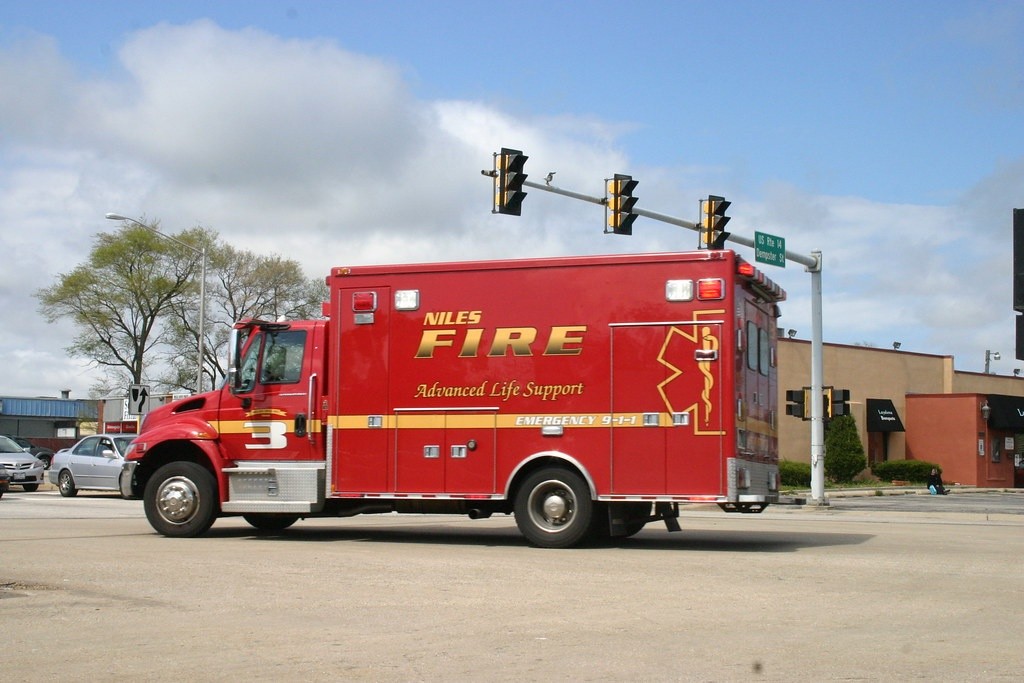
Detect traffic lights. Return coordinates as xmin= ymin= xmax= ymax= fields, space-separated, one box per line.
xmin=784 ymin=389 xmax=804 ymax=419
xmin=610 ymin=173 xmax=640 ymax=235
xmin=703 ymin=194 xmax=731 ymax=248
xmin=832 ymin=389 xmax=850 ymax=418
xmin=500 ymin=148 xmax=529 ymax=215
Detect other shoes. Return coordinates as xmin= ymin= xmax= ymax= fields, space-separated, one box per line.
xmin=943 ymin=490 xmax=950 ymax=494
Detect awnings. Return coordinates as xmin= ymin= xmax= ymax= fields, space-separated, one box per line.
xmin=866 ymin=398 xmax=906 ymax=432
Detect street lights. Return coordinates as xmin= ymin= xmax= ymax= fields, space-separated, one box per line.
xmin=105 ymin=213 xmax=205 ymax=395
xmin=985 ymin=352 xmax=1000 ymax=373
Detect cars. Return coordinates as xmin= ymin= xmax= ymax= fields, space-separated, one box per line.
xmin=5 ymin=435 xmax=56 ymax=469
xmin=48 ymin=434 xmax=139 ymax=499
xmin=0 ymin=435 xmax=46 ymax=493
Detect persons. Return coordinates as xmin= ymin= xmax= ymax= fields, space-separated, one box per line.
xmin=928 ymin=468 xmax=950 ymax=495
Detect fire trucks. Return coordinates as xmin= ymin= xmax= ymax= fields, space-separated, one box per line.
xmin=118 ymin=249 xmax=787 ymax=546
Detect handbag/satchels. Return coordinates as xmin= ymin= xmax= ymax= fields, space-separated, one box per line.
xmin=929 ymin=485 xmax=937 ymax=494
